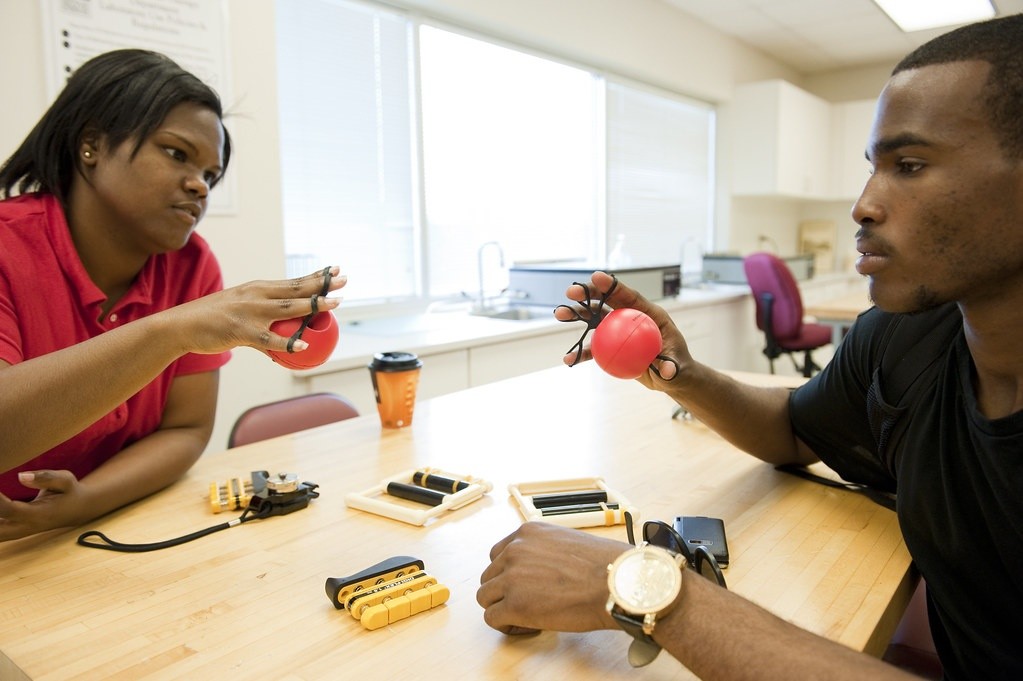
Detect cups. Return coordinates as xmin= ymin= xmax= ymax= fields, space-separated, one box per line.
xmin=368 ymin=351 xmax=424 ymax=430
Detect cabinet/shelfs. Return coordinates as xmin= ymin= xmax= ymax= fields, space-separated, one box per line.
xmin=732 ymin=80 xmax=883 ymax=198
xmin=309 ymin=302 xmax=740 ymax=416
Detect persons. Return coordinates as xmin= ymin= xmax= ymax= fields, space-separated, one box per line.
xmin=477 ymin=9 xmax=1023 ymax=679
xmin=0 ymin=48 xmax=347 ymax=542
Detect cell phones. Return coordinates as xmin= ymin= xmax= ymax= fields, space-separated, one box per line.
xmin=672 ymin=516 xmax=729 ymax=569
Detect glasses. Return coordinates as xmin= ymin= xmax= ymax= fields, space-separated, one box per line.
xmin=625 ymin=511 xmax=728 ymax=590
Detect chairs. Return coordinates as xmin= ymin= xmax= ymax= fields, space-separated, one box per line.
xmin=744 ymin=253 xmax=833 ymax=378
xmin=229 ymin=391 xmax=360 ymax=446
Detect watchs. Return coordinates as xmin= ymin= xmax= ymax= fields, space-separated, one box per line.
xmin=605 ymin=541 xmax=689 ymax=668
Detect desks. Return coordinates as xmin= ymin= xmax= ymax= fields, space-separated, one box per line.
xmin=0 ymin=359 xmax=921 ymax=681
xmin=792 ymin=279 xmax=874 ymax=318
